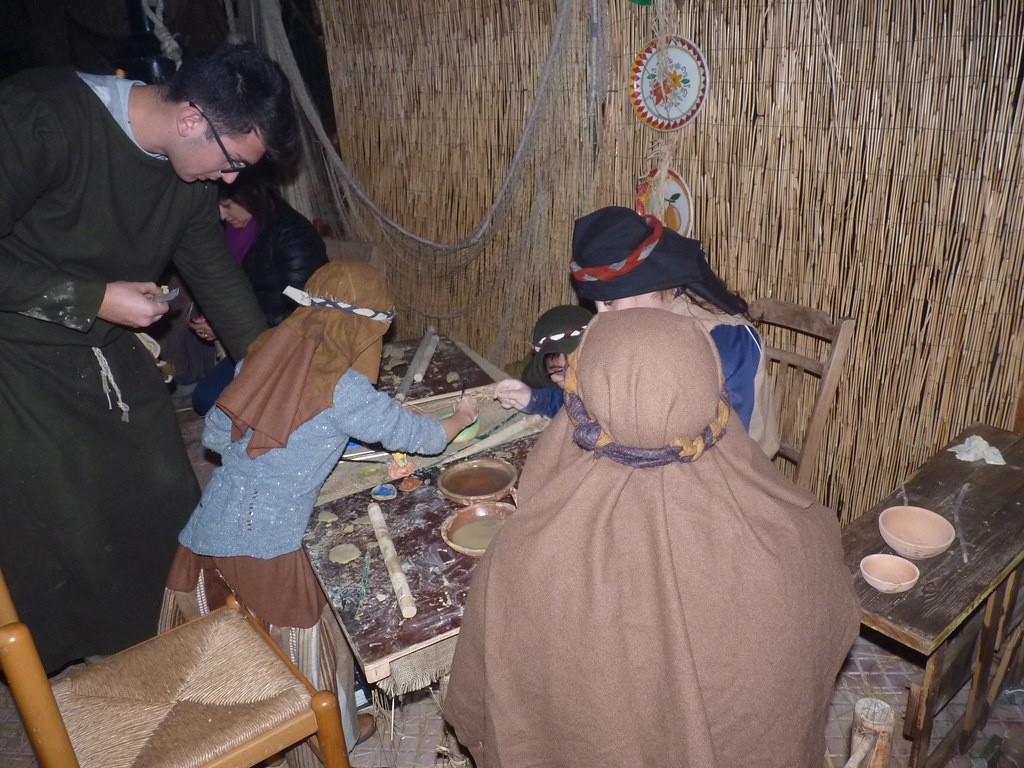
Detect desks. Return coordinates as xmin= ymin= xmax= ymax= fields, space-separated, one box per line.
xmin=839 ymin=421 xmax=1024 ymax=768
xmin=299 ymin=337 xmax=552 ymax=709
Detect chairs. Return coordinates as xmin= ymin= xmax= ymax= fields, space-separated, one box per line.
xmin=0 ymin=576 xmax=352 ymax=768
xmin=748 ymin=299 xmax=857 ymax=488
xmin=215 ymin=238 xmax=384 ymax=360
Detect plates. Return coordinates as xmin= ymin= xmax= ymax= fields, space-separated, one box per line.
xmin=637 ymin=168 xmax=694 ymax=238
xmin=629 ymin=35 xmax=709 ymax=131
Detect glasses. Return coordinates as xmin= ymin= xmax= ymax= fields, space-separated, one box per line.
xmin=544 ymin=369 xmax=566 ymax=383
xmin=189 ymin=102 xmax=249 ymax=173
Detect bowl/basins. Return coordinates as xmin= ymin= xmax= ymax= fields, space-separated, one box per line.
xmin=439 ymin=413 xmax=480 ymax=442
xmin=860 ymin=554 xmax=920 ymax=594
xmin=878 ymin=506 xmax=956 ymax=561
xmin=441 ymin=501 xmax=517 ymax=557
xmin=438 ymin=457 xmax=518 ymax=506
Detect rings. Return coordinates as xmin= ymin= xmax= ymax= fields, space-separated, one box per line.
xmin=203 ymin=333 xmax=208 ymax=337
xmin=203 ymin=317 xmax=206 ymax=321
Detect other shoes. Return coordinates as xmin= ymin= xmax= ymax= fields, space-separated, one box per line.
xmin=354 ymin=712 xmax=376 ymax=746
xmin=266 ymin=752 xmax=284 ymax=766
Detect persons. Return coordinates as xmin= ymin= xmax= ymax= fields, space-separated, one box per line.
xmin=158 ymin=259 xmax=479 ymax=768
xmin=569 ymin=206 xmax=780 ymax=461
xmin=443 ymin=307 xmax=862 ymax=768
xmin=188 ymin=170 xmax=330 ymax=416
xmin=1 ymin=47 xmax=303 ymax=683
xmin=493 ymin=305 xmax=593 ymax=419
